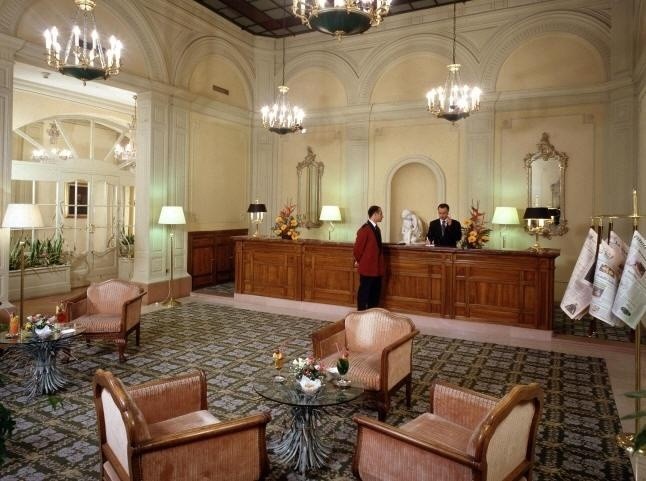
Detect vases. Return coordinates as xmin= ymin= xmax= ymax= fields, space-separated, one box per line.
xmin=33 ymin=325 xmax=51 ymax=338
xmin=298 ymin=374 xmax=321 ymax=391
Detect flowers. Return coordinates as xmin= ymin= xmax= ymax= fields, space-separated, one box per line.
xmin=289 ymin=356 xmax=328 ymax=378
xmin=458 ymin=199 xmax=492 ymax=250
xmin=24 ymin=310 xmax=57 ymax=337
xmin=270 ymin=199 xmax=303 ymax=240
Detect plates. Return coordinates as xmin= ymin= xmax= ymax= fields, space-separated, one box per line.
xmin=412 ymin=243 xmax=426 ymax=247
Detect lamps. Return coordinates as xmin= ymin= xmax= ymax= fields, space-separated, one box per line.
xmin=490 ymin=204 xmax=520 ymax=250
xmin=39 ymin=0 xmax=126 ymax=88
xmin=155 ymin=205 xmax=191 ymax=309
xmin=256 ymin=25 xmax=308 ymax=136
xmin=289 ymin=0 xmax=399 ymax=39
xmin=246 ymin=202 xmax=268 ymax=238
xmin=524 ymin=205 xmax=553 ymax=252
xmin=417 ymin=0 xmax=485 ymax=127
xmin=30 ymin=117 xmax=74 ymax=165
xmin=319 ymin=205 xmax=343 ymax=240
xmin=111 ymin=94 xmax=141 ymax=169
xmin=1 ymin=201 xmax=49 ymax=328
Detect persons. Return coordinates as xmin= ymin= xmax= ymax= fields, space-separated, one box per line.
xmin=428 ymin=203 xmax=461 ymax=248
xmin=352 ymin=203 xmax=386 ymax=312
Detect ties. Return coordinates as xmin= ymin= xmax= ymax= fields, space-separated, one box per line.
xmin=441 ymin=221 xmax=446 ymax=237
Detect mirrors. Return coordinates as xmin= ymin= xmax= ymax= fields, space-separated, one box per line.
xmin=295 ymin=145 xmax=325 ymax=231
xmin=525 ymin=131 xmax=569 ymax=243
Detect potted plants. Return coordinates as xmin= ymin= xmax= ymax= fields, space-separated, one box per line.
xmin=117 ymin=231 xmax=134 ymax=281
xmin=6 ymin=233 xmax=70 ymax=304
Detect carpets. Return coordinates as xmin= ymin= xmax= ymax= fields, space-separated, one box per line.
xmin=553 ymin=301 xmax=644 ymax=350
xmin=193 ymin=277 xmax=234 ymax=297
xmin=1 ymin=297 xmax=638 ymax=480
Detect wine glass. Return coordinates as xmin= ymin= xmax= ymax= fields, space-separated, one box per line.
xmin=335 ymin=356 xmax=352 ymax=389
xmin=270 ymin=353 xmax=285 ymax=383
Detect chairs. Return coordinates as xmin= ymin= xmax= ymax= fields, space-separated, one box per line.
xmin=305 ymin=305 xmax=425 ymax=423
xmin=348 ymin=365 xmax=545 ymax=481
xmin=52 ymin=278 xmax=147 ymax=368
xmin=84 ymin=362 xmax=272 ymax=480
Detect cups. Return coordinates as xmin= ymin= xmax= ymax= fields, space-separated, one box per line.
xmin=9 ymin=316 xmax=20 ymax=337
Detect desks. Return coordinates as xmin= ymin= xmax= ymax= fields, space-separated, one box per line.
xmin=2 ymin=322 xmax=88 ymax=402
xmin=244 ymin=361 xmax=370 ymax=477
